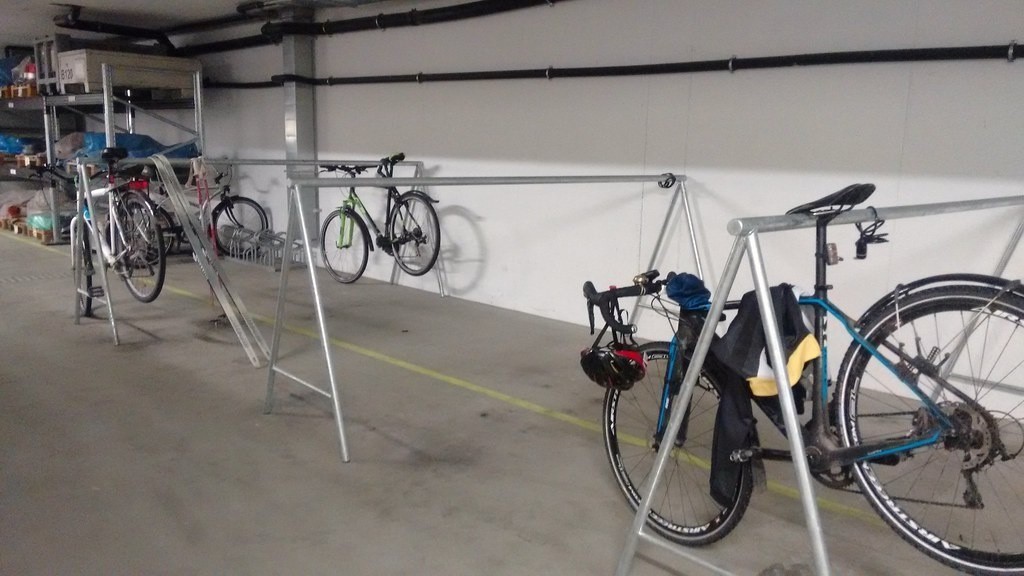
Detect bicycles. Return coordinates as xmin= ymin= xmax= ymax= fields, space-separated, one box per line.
xmin=107 ymin=155 xmax=270 ymax=269
xmin=27 ymin=146 xmax=166 ymax=315
xmin=582 ymin=184 xmax=1024 ymax=576
xmin=318 ymin=153 xmax=442 ymax=283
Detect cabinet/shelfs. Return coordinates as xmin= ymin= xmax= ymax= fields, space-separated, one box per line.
xmin=0 ymin=65 xmax=211 ymax=244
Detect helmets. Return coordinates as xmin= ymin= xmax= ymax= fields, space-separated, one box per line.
xmin=580 ymin=343 xmax=648 ymax=391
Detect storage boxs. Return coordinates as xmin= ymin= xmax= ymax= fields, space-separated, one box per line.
xmin=17 ymin=153 xmax=37 ymax=168
xmin=2 ymin=218 xmax=53 ymax=241
xmin=66 ymin=162 xmax=97 ymax=177
xmin=57 ymin=49 xmax=202 ymax=98
xmin=0 ymin=85 xmax=36 ymax=99
